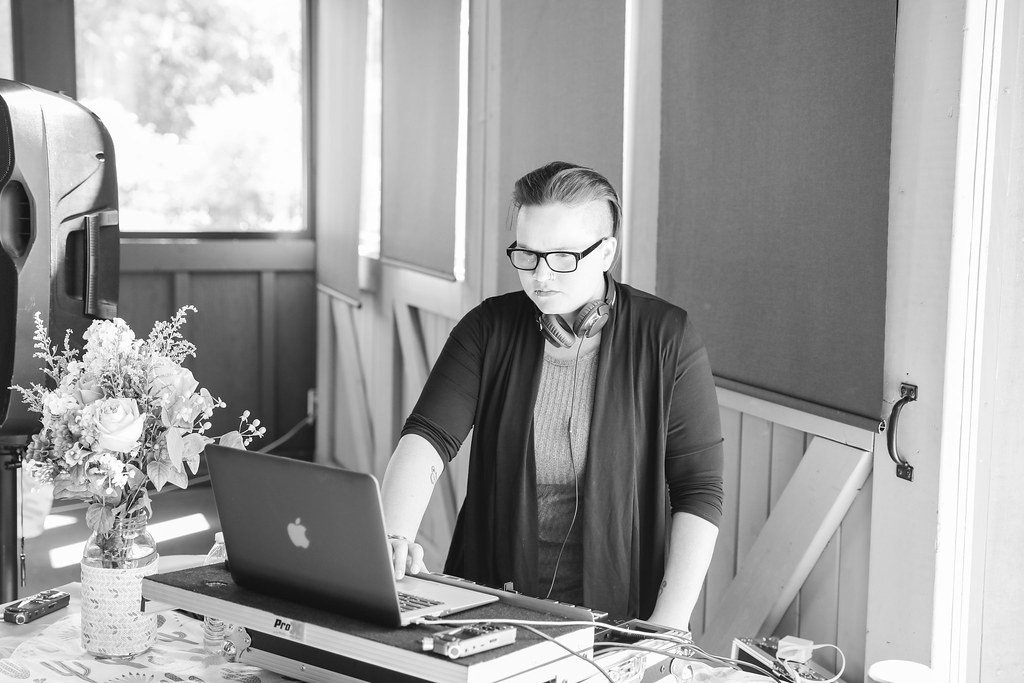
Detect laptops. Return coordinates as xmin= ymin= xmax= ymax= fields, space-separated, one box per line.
xmin=205 ymin=443 xmax=499 ymax=627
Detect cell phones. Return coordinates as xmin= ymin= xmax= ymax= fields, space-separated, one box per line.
xmin=426 ymin=623 xmax=516 ymax=660
xmin=4 ymin=591 xmax=70 ymax=624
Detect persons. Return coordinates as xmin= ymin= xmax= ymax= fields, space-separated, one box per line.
xmin=381 ymin=161 xmax=723 ymax=633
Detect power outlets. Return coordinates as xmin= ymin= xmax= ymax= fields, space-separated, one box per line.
xmin=307 ymin=389 xmax=318 ymax=422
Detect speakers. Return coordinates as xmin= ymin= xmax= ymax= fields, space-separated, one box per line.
xmin=0 ymin=77 xmax=120 ymax=441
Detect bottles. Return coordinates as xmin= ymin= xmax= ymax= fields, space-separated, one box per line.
xmin=201 ymin=532 xmax=230 ymax=654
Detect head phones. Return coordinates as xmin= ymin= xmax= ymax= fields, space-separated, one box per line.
xmin=534 ymin=270 xmax=616 ymax=348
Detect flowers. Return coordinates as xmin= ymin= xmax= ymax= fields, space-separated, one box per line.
xmin=8 ymin=302 xmax=264 ymax=533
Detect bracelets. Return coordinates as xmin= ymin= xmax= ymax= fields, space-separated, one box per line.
xmin=387 ymin=533 xmax=406 ymax=539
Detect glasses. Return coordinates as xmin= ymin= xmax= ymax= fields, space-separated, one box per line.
xmin=506 ymin=237 xmax=607 ymax=273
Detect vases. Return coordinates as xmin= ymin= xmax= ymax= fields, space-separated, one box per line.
xmin=81 ymin=510 xmax=160 ymax=659
